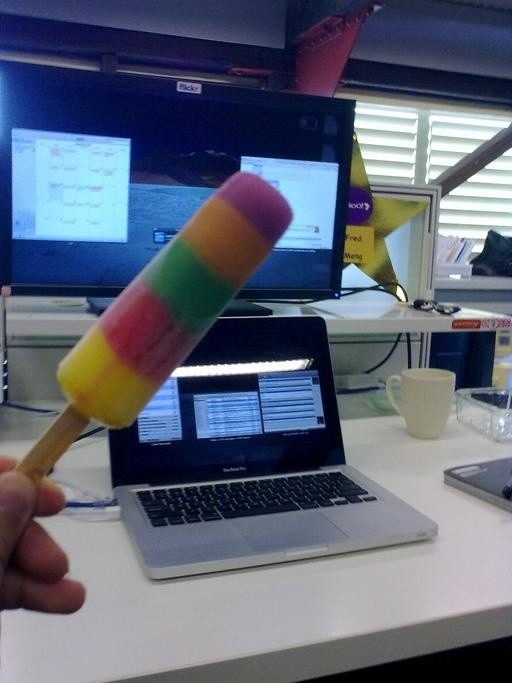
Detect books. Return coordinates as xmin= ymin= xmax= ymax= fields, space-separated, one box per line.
xmin=443 ymin=457 xmax=512 ymax=514
xmin=435 ymin=234 xmax=477 ymax=264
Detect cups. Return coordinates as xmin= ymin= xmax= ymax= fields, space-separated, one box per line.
xmin=387 ymin=367 xmax=459 ymax=438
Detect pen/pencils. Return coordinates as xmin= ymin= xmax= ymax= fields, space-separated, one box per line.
xmin=502 ymin=471 xmax=512 ymax=496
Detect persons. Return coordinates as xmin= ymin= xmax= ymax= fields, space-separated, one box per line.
xmin=1 ymin=458 xmax=86 ymax=615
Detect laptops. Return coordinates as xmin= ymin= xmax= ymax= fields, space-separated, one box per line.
xmin=108 ymin=314 xmax=441 ymax=581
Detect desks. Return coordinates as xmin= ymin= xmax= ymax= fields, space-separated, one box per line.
xmin=1 ymin=289 xmax=512 ymax=683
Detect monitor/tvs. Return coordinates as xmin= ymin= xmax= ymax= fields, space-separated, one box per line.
xmin=1 ymin=57 xmax=357 ymax=315
